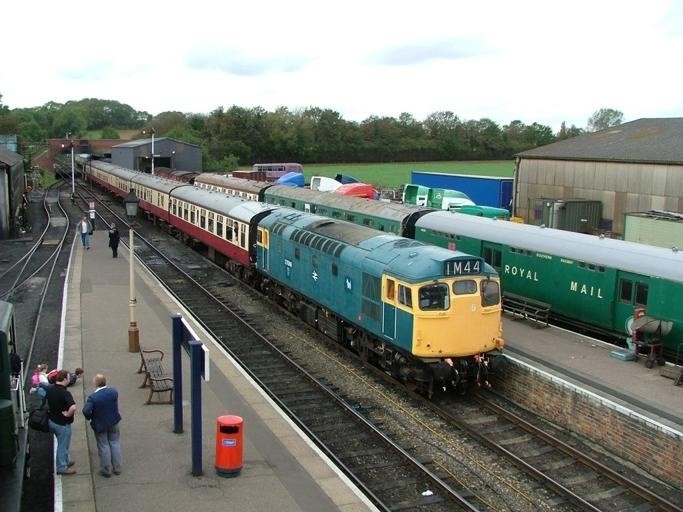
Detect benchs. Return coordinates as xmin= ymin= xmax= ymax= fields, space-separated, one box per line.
xmin=137 ymin=344 xmax=174 ymax=405
xmin=501 ymin=291 xmax=552 ymax=329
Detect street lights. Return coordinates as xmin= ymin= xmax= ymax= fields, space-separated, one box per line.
xmin=124 ymin=188 xmax=144 ymax=353
xmin=70 ymin=141 xmax=78 ymax=205
xmin=149 ymin=128 xmax=160 ymax=177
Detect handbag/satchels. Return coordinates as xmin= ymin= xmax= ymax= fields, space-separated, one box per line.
xmin=28 ymin=408 xmax=50 ymax=433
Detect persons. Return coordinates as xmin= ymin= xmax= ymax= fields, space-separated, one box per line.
xmin=106 ymin=222 xmax=119 ymax=259
xmin=75 ymin=215 xmax=93 ymax=249
xmin=32 ymin=363 xmax=49 ymax=412
xmin=42 ymin=369 xmax=77 ymax=478
xmin=25 ymin=367 xmax=83 ymax=396
xmin=80 ymin=373 xmax=122 ymax=479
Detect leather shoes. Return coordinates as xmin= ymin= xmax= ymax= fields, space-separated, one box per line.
xmin=57 ymin=461 xmax=77 ymax=474
xmin=99 ymin=470 xmax=121 ymax=477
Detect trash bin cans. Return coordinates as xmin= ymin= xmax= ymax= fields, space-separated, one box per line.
xmin=214 ymin=414 xmax=243 ymax=478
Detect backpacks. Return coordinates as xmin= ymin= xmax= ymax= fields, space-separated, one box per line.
xmin=47 ymin=369 xmax=59 ymax=384
xmin=32 ymin=372 xmax=39 ymax=385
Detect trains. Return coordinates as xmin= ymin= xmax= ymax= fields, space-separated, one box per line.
xmin=81 ymin=160 xmax=509 ymax=401
xmin=0 ymin=299 xmax=29 ymax=468
xmin=146 ymin=164 xmax=683 ymax=369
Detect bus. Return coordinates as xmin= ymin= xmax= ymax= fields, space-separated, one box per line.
xmin=251 ymin=162 xmax=304 ymax=183
